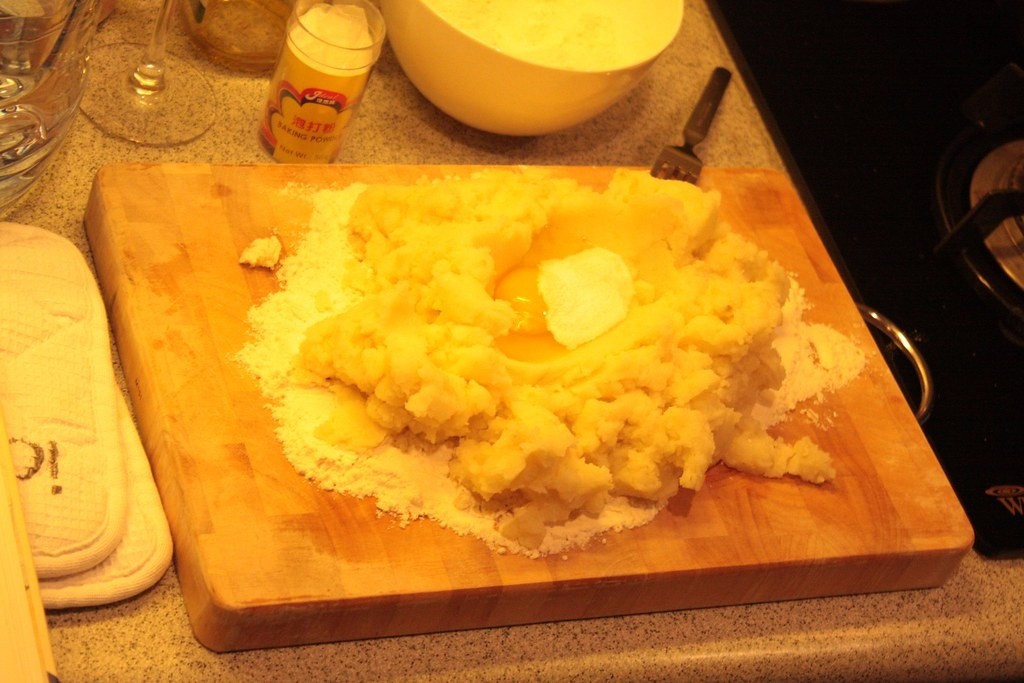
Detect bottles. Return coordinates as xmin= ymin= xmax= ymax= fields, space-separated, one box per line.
xmin=257 ymin=1 xmax=387 ymax=168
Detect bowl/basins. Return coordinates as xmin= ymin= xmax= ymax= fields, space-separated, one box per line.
xmin=382 ymin=0 xmax=683 ymax=138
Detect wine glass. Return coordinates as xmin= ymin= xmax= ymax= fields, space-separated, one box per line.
xmin=78 ymin=1 xmax=221 ymax=147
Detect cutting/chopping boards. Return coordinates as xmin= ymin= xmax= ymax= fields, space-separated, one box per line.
xmin=83 ymin=160 xmax=975 ymax=654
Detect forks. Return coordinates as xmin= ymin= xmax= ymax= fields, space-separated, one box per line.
xmin=649 ymin=67 xmax=731 ymax=182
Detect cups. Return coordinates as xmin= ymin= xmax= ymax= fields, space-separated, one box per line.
xmin=0 ymin=1 xmax=99 ymax=220
xmin=175 ymin=1 xmax=293 ymax=74
